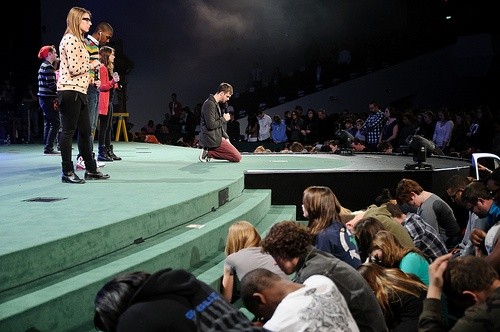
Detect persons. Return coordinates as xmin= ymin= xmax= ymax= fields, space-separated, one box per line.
xmin=199 ymin=83 xmax=242 ymax=163
xmin=0 ymin=7 xmax=500 ymax=332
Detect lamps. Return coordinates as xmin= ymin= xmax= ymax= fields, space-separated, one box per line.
xmin=404 ymin=134 xmax=436 ymax=171
xmin=335 ymin=130 xmax=355 ymax=155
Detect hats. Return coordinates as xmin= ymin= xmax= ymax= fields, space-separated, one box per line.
xmin=227 ymin=106 xmax=234 ymax=113
xmin=38 ymin=46 xmax=51 ymax=59
xmin=485 ymin=224 xmax=500 ymax=256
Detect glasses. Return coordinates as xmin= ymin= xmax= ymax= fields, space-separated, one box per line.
xmin=450 ymin=189 xmax=460 ymax=203
xmin=82 ymin=18 xmax=92 ymax=21
xmin=466 ymin=204 xmax=475 ymax=215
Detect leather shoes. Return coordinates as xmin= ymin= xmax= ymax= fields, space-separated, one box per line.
xmin=62 ymin=170 xmax=86 ymax=184
xmin=85 ymin=170 xmax=110 ymax=180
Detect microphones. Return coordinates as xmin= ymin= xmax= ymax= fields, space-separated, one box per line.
xmin=94 ymin=66 xmax=99 ymax=88
xmin=225 ymin=102 xmax=228 ymax=113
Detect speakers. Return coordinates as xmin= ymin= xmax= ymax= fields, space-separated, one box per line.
xmin=413 ymin=137 xmax=428 ymax=162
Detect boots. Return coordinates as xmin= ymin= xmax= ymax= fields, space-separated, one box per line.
xmin=97 ymin=144 xmax=113 ymax=161
xmin=105 ymin=145 xmax=121 ymax=160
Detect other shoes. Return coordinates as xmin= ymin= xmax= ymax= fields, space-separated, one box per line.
xmin=199 ymin=148 xmax=210 ymax=163
xmin=57 ymin=145 xmax=74 ymax=151
xmin=96 ymin=162 xmax=105 ymax=168
xmin=76 ymin=156 xmax=86 ymax=171
xmin=43 ymin=150 xmax=61 ymax=155
